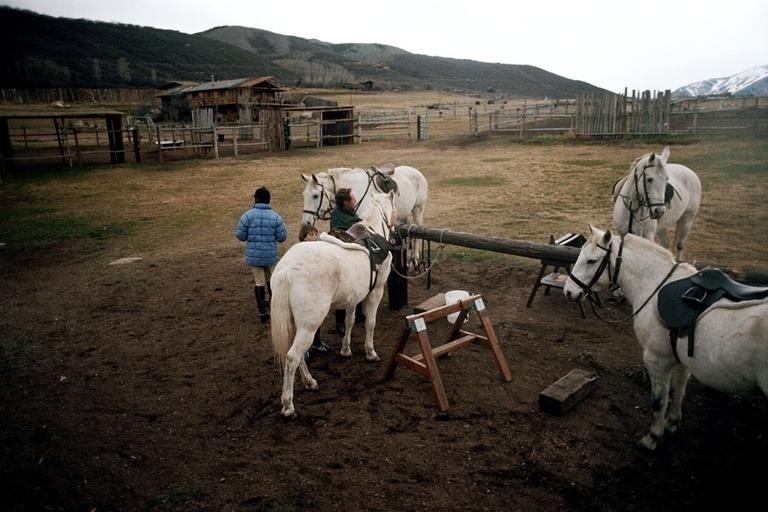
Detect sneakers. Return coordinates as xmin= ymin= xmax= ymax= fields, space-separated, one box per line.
xmin=312 ymin=343 xmax=331 ymax=352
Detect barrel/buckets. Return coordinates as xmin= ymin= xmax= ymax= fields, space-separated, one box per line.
xmin=446 ymin=290 xmax=471 ymax=324
xmin=218 ymin=134 xmax=225 ymax=141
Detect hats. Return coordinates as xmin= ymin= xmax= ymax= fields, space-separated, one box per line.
xmin=252 ymin=187 xmax=270 ymax=201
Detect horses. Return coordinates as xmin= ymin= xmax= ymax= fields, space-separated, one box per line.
xmin=613 ymin=146 xmax=702 ymax=297
xmin=270 ymin=189 xmax=398 ymax=419
xmin=300 ymin=166 xmax=429 ymax=274
xmin=563 ymin=223 xmax=768 ymax=452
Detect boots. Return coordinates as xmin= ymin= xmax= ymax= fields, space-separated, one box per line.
xmin=255 ymin=286 xmax=269 ymax=322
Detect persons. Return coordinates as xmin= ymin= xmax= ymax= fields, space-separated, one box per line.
xmin=330 ymin=187 xmax=366 ymax=336
xmin=299 ymin=226 xmax=332 ymax=354
xmin=235 ymin=186 xmax=288 ymax=324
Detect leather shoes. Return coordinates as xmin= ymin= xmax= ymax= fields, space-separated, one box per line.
xmin=338 ymin=322 xmax=345 ymax=334
